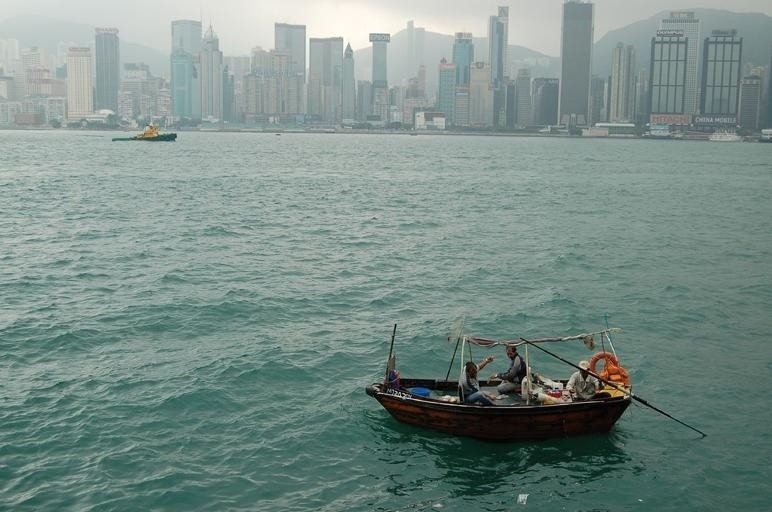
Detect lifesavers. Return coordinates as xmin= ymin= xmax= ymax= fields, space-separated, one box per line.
xmin=601 ymin=367 xmax=630 ymax=385
xmin=590 ymin=352 xmax=619 ymax=373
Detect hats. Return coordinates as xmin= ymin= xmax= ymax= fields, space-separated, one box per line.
xmin=579 ymin=360 xmax=590 ymax=370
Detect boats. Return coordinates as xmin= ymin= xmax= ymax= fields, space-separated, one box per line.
xmin=705 ymin=125 xmax=742 ymax=142
xmin=111 ymin=120 xmax=178 ymax=143
xmin=366 ymin=313 xmax=632 ymax=443
xmin=758 ymin=126 xmax=772 ymax=142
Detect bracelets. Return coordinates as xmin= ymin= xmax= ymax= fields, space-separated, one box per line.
xmin=485 ymin=358 xmax=490 ymax=362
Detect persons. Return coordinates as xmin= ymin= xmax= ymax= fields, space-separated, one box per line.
xmin=459 ymin=355 xmax=498 ymax=405
xmin=491 ymin=346 xmax=526 ymax=395
xmin=565 ymin=360 xmax=604 ymax=401
